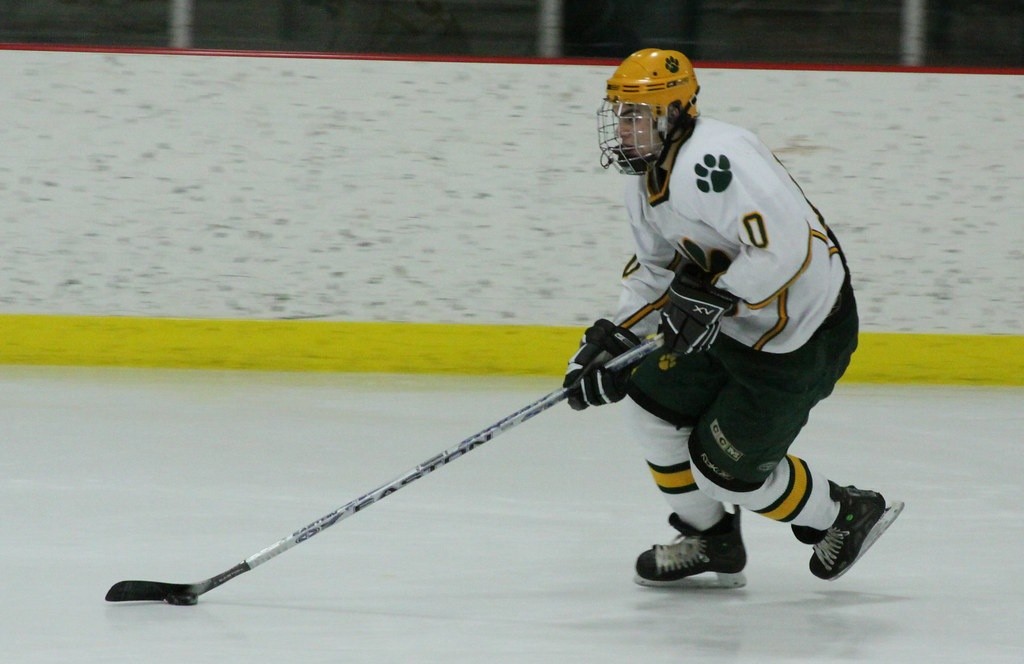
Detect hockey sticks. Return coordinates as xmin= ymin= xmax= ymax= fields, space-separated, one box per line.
xmin=105 ymin=333 xmax=668 ymax=607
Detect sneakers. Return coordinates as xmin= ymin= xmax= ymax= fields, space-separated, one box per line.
xmin=790 ymin=479 xmax=905 ymax=582
xmin=633 ymin=503 xmax=747 ymax=588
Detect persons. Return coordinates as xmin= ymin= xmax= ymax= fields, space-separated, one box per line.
xmin=562 ymin=47 xmax=904 ymax=589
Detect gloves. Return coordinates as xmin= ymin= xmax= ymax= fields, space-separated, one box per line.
xmin=656 ymin=272 xmax=741 ymax=357
xmin=563 ymin=318 xmax=649 ymax=411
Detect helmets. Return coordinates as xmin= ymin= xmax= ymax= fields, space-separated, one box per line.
xmin=596 ymin=48 xmax=701 ymax=176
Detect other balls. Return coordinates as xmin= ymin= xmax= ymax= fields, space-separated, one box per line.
xmin=166 ymin=591 xmax=199 ymax=606
